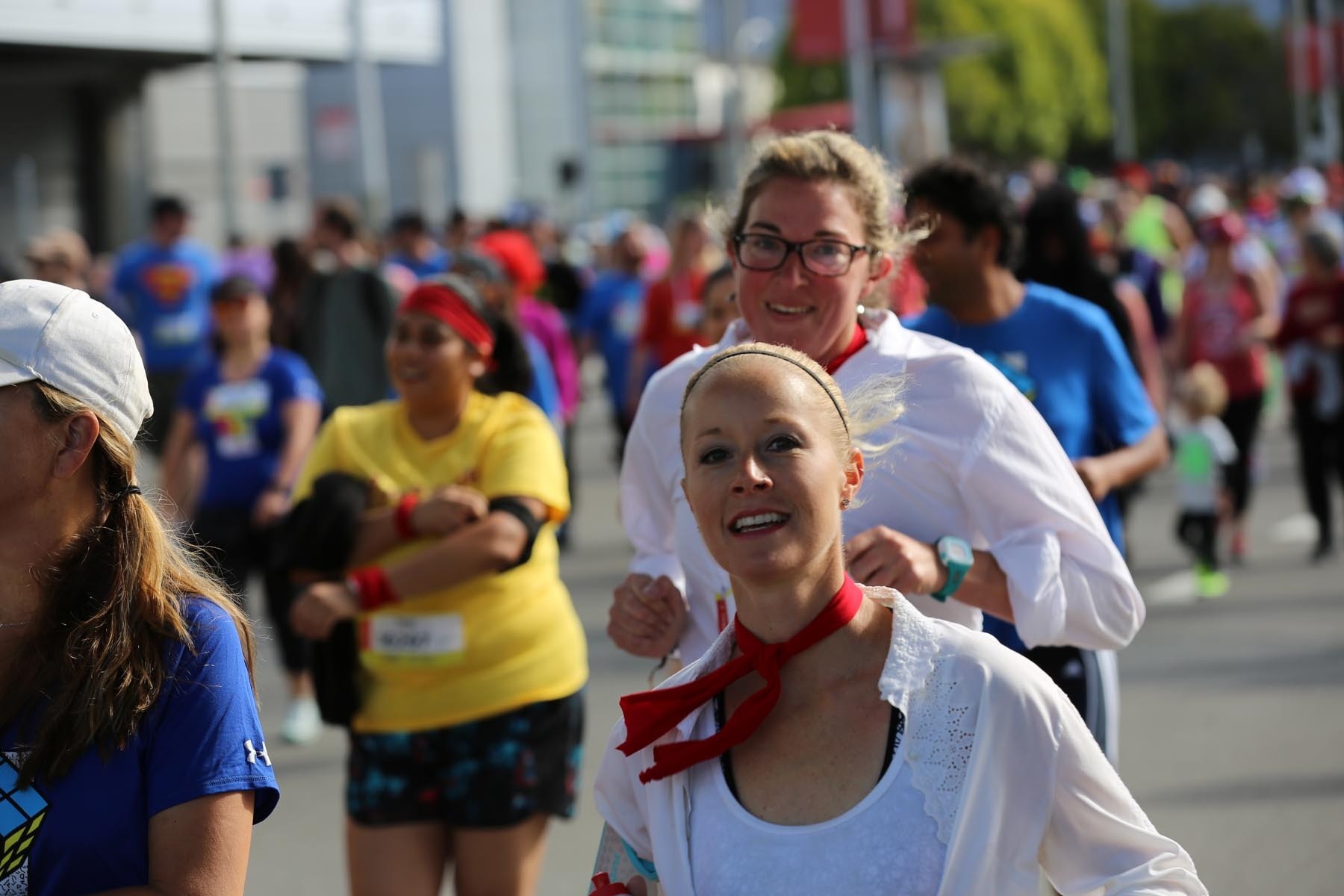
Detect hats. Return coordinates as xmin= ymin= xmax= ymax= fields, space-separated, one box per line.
xmin=0 ymin=279 xmax=157 ymax=446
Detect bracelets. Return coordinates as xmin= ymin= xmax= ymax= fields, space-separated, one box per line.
xmin=343 ymin=562 xmax=400 ymax=613
xmin=393 ymin=494 xmax=417 ymax=543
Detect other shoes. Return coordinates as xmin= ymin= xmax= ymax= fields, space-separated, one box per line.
xmin=281 ymin=696 xmax=326 ymax=744
xmin=1194 ymin=571 xmax=1231 ymax=598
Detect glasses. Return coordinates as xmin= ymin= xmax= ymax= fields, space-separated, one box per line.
xmin=732 ymin=232 xmax=874 ymax=277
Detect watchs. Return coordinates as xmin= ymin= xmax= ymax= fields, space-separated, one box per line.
xmin=930 ymin=534 xmax=974 ymax=603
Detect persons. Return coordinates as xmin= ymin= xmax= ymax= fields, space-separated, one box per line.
xmin=0 ymin=279 xmax=280 ymax=896
xmin=608 ymin=129 xmax=1145 ymax=697
xmin=588 ymin=336 xmax=1207 ymax=896
xmin=291 ymin=274 xmax=589 ymax=896
xmin=858 ymin=152 xmax=1344 ymax=769
xmin=20 ymin=193 xmax=742 ymax=744
